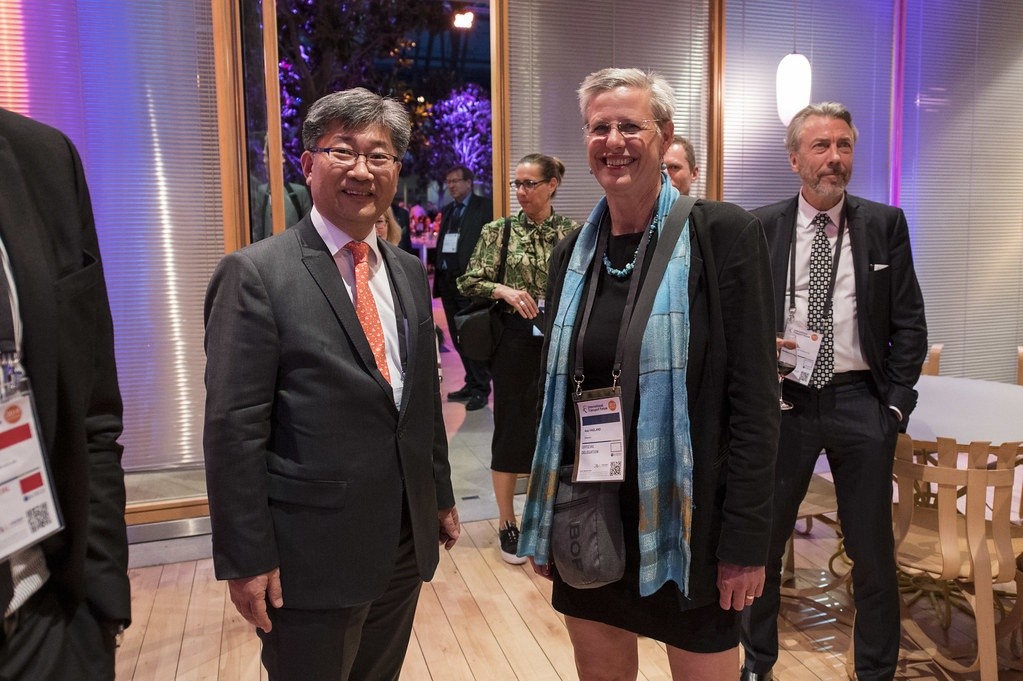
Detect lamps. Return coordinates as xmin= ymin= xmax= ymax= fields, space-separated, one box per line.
xmin=775 ymin=0 xmax=813 ymax=127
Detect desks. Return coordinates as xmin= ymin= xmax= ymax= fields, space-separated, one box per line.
xmin=813 ymin=375 xmax=1021 ymax=631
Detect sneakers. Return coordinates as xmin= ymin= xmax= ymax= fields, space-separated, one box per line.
xmin=498 ymin=520 xmax=528 ymax=565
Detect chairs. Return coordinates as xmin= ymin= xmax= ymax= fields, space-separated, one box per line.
xmin=776 ymin=344 xmax=1023 ymax=681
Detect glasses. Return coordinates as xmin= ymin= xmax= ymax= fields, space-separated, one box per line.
xmin=375 ymin=218 xmax=389 ymax=230
xmin=581 ymin=118 xmax=661 ymax=137
xmin=308 ymin=146 xmax=399 ymax=165
xmin=446 ymin=177 xmax=465 ymax=184
xmin=510 ymin=178 xmax=548 ymax=192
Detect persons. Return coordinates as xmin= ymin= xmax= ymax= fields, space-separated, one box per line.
xmin=431 ymin=164 xmax=496 ymax=412
xmin=0 ymin=103 xmax=134 ymax=680
xmin=664 ymin=133 xmax=699 ymax=197
xmin=241 ymin=141 xmax=452 ymax=386
xmin=200 ymin=88 xmax=461 ymax=680
xmin=454 ymin=153 xmax=588 ymax=566
xmin=741 ymin=101 xmax=929 ymax=681
xmin=512 ymin=67 xmax=777 ymax=680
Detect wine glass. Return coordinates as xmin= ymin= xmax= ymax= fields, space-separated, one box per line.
xmin=776 ymin=332 xmax=797 ymax=410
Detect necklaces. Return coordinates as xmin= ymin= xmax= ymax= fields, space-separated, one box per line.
xmin=600 ymin=211 xmax=659 ymax=277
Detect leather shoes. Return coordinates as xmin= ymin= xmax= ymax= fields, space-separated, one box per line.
xmin=740 ymin=665 xmax=774 ymax=681
xmin=465 ymin=396 xmax=489 ymax=410
xmin=447 ymin=382 xmax=477 ymax=402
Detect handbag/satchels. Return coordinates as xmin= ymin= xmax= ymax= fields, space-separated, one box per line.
xmin=453 ymin=297 xmax=504 ymax=365
xmin=548 ymin=459 xmax=627 ymax=590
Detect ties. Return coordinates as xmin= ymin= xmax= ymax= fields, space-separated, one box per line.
xmin=807 ymin=213 xmax=833 ymax=389
xmin=445 ymin=203 xmax=464 ymax=268
xmin=345 ymin=241 xmax=391 ymax=384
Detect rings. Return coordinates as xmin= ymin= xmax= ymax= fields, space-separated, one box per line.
xmin=746 ymin=595 xmax=755 ymax=600
xmin=519 ymin=301 xmax=525 ymax=306
